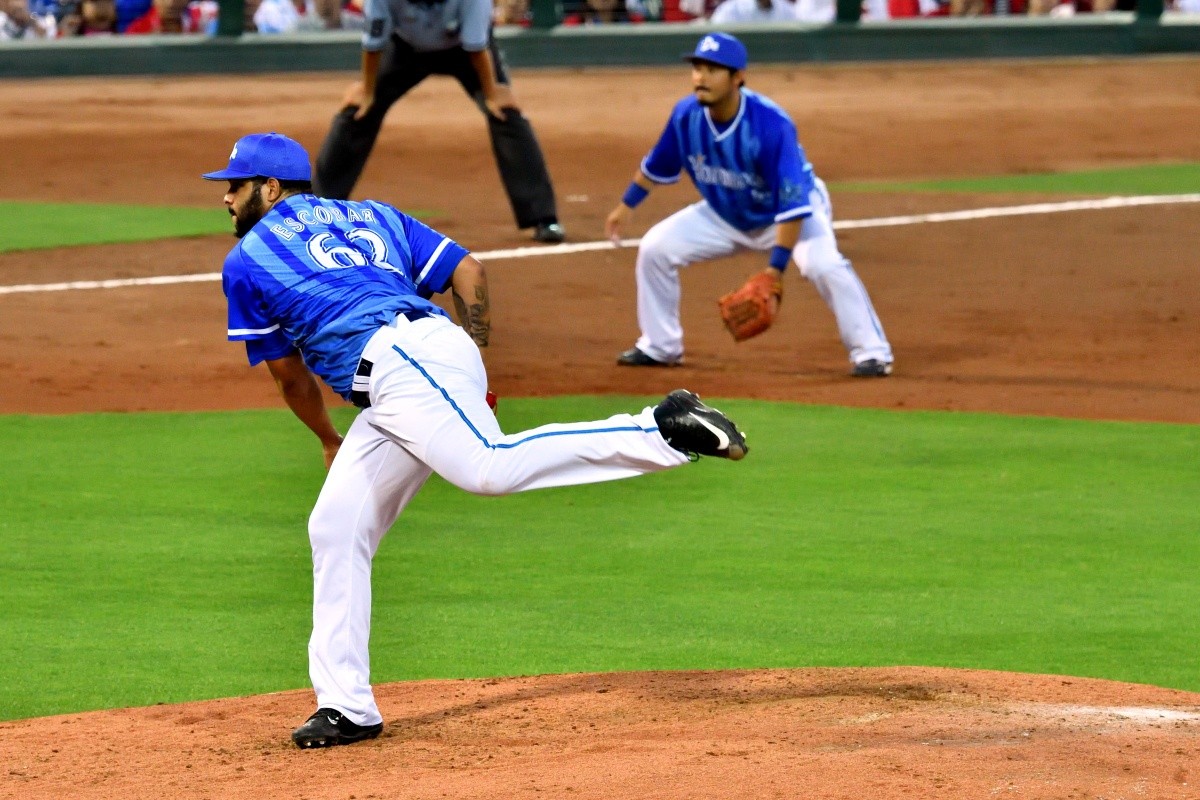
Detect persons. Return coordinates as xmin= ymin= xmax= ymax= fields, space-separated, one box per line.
xmin=0 ymin=0 xmax=364 ymax=42
xmin=489 ymin=0 xmax=1200 ymax=27
xmin=603 ymin=31 xmax=894 ymax=379
xmin=310 ymin=0 xmax=564 ymax=242
xmin=200 ymin=132 xmax=748 ymax=750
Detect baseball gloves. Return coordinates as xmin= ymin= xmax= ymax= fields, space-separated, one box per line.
xmin=720 ymin=272 xmax=783 ymax=342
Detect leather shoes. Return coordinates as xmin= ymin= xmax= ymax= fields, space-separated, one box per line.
xmin=352 ymin=308 xmax=431 ymax=408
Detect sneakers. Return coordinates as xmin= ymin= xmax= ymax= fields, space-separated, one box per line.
xmin=535 ymin=223 xmax=564 ymax=239
xmin=654 ymin=386 xmax=748 ymax=461
xmin=854 ymin=360 xmax=891 ymax=376
xmin=293 ymin=708 xmax=384 ymax=748
xmin=619 ymin=346 xmax=686 ymax=367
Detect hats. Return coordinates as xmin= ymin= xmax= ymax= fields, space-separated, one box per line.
xmin=202 ymin=131 xmax=311 ymax=181
xmin=684 ymin=32 xmax=747 ymax=71
